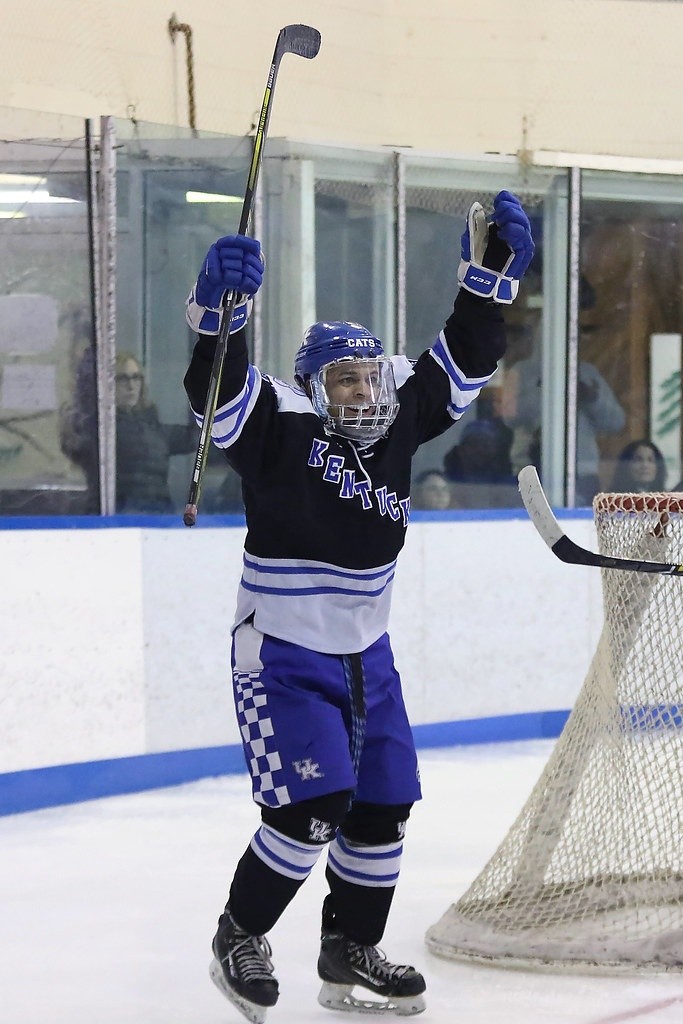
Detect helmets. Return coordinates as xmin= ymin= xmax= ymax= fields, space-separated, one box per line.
xmin=293 ymin=319 xmax=400 ymax=444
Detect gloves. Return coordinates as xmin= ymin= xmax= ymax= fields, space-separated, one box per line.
xmin=185 ymin=235 xmax=266 ymax=334
xmin=456 ymin=189 xmax=535 ymax=305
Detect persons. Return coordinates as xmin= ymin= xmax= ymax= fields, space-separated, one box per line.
xmin=63 ymin=354 xmax=214 ymax=512
xmin=185 ymin=190 xmax=535 ymax=1024
xmin=410 ymin=356 xmax=667 ymax=511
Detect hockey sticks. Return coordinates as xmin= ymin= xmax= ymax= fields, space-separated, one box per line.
xmin=513 ymin=462 xmax=682 ymax=579
xmin=178 ymin=21 xmax=327 ymax=531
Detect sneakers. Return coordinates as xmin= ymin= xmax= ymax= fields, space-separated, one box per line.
xmin=208 ymin=900 xmax=280 ymax=1024
xmin=317 ymin=893 xmax=427 ymax=1015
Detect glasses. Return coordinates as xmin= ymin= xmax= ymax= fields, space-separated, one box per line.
xmin=115 ymin=372 xmax=145 ymax=385
